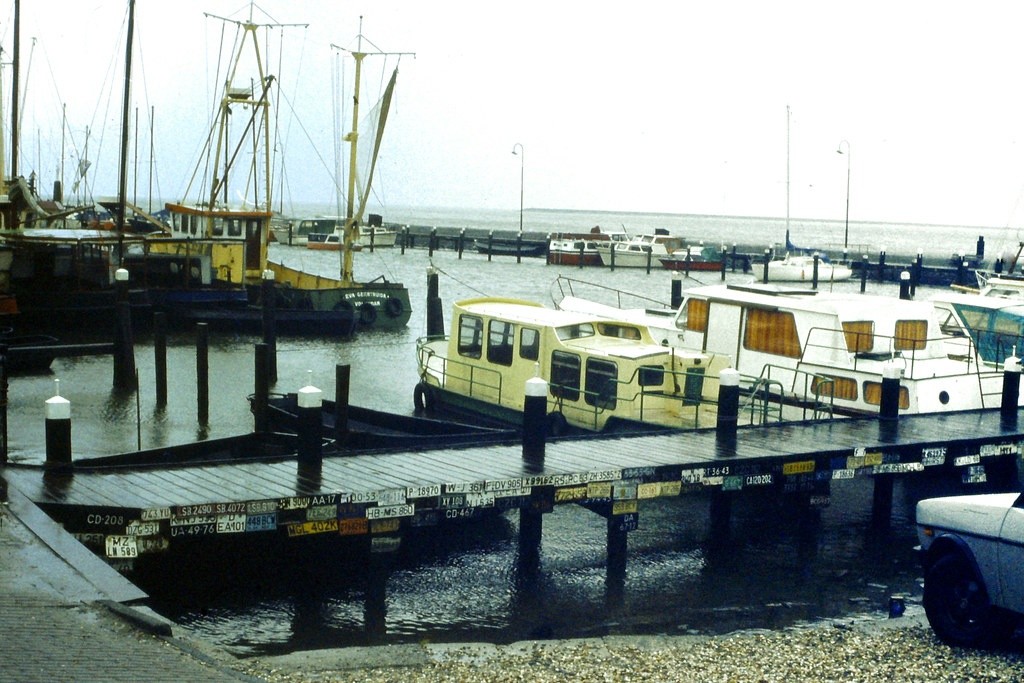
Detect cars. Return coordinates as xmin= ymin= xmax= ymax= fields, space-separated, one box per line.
xmin=916 ymin=489 xmax=1024 ymax=648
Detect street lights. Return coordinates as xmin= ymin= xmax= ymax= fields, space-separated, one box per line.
xmin=512 ymin=144 xmax=524 ymax=237
xmin=836 ymin=141 xmax=852 ymax=260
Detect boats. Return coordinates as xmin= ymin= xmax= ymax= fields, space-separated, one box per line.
xmin=545 ymin=225 xmax=728 ymax=272
xmin=550 ymin=229 xmax=1024 ymax=418
xmin=474 ymin=237 xmax=545 ymax=258
xmin=272 ymin=214 xmax=400 ymax=250
xmin=416 ymin=267 xmax=835 ymax=435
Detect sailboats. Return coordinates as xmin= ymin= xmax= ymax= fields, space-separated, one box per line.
xmin=0 ymin=0 xmax=417 ymax=335
xmin=751 ymin=101 xmax=853 ymax=283
